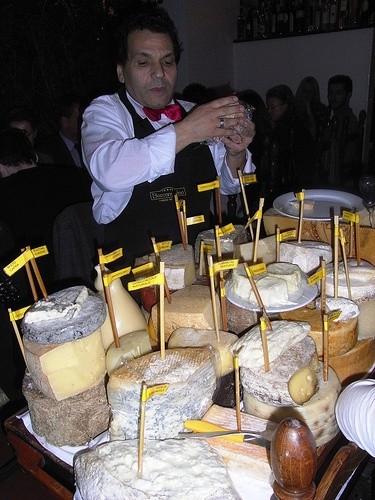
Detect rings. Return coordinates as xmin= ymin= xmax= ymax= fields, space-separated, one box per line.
xmin=237 ymin=137 xmax=243 ymax=144
xmin=219 ymin=117 xmax=224 ymax=129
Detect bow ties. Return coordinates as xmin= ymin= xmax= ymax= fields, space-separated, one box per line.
xmin=142 ymin=104 xmax=182 ymax=123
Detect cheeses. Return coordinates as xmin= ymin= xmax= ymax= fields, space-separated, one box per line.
xmin=22 ymin=205 xmax=375 ymax=500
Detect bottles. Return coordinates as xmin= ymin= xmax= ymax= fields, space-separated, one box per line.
xmin=94 ymin=264 xmax=148 ymax=350
xmin=237 ymin=0 xmax=375 ymax=40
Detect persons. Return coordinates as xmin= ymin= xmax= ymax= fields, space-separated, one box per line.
xmin=81 ymin=11 xmax=255 ymax=309
xmin=183 ymin=75 xmax=362 ymax=219
xmin=334 ymin=379 xmax=375 ymax=458
xmin=0 ymin=99 xmax=86 ymax=412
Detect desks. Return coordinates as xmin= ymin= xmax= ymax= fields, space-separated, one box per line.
xmin=4 ymin=392 xmax=367 ymax=500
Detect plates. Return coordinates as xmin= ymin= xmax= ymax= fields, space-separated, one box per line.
xmin=272 ymin=187 xmax=365 ymax=221
xmin=225 ymin=275 xmax=318 ymax=312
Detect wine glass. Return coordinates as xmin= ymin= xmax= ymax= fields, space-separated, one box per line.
xmin=359 ymin=177 xmax=375 ymax=228
